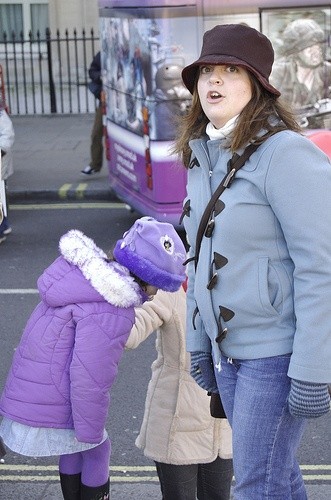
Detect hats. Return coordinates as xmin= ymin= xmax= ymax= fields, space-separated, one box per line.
xmin=112 ymin=217 xmax=187 ymax=293
xmin=181 ymin=24 xmax=281 ymax=98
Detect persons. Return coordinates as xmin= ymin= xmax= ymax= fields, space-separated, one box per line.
xmin=0 ymin=98 xmax=15 ymax=243
xmin=108 ymin=46 xmax=150 ymax=126
xmin=1 ymin=216 xmax=187 ymax=500
xmin=269 ymin=18 xmax=331 ymax=130
xmin=125 ymin=226 xmax=234 ymax=500
xmin=155 ymin=63 xmax=191 ymax=117
xmin=178 ymin=23 xmax=331 ymax=500
xmin=80 ymin=49 xmax=103 ymax=175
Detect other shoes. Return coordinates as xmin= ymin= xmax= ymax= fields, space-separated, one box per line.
xmin=80 ymin=166 xmax=99 ymax=175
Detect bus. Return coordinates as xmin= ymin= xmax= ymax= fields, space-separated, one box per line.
xmin=97 ymin=1 xmax=331 ymax=229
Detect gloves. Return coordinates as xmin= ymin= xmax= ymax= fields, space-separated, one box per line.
xmin=189 ymin=351 xmax=219 ymax=394
xmin=285 ymin=378 xmax=331 ymax=420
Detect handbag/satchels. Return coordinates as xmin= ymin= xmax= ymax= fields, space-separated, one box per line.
xmin=208 ymin=392 xmax=226 ymax=419
